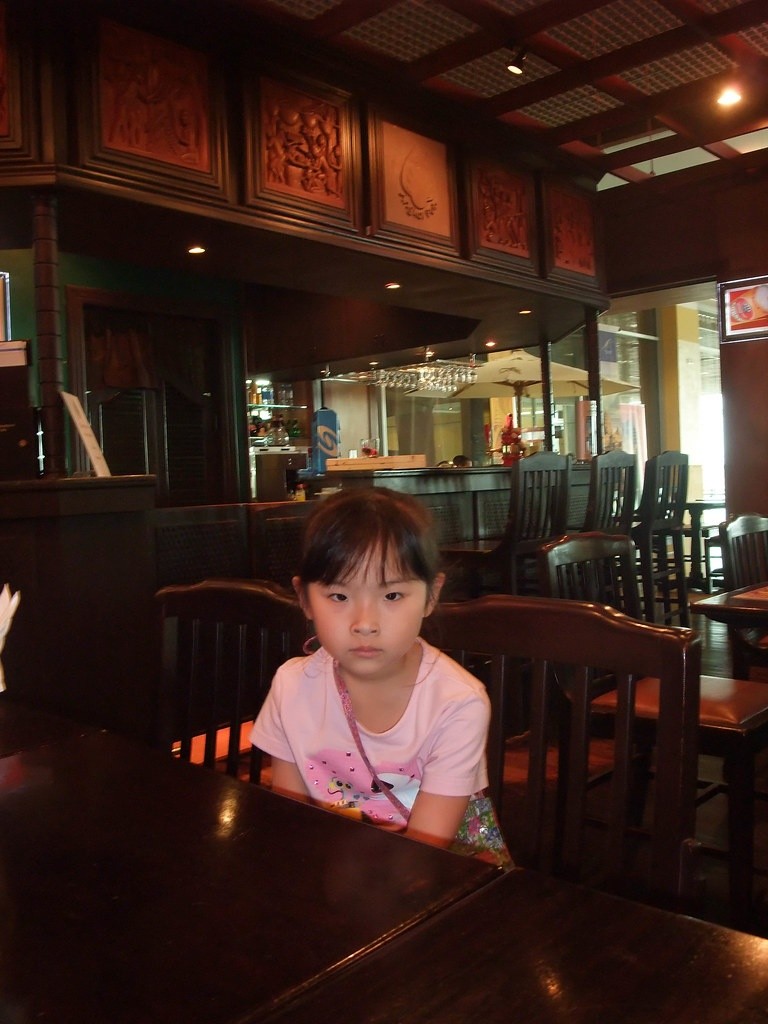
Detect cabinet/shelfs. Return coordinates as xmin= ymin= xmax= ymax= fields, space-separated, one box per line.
xmin=246 ymin=403 xmax=311 ymax=441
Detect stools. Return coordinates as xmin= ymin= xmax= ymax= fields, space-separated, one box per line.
xmin=439 ymin=449 xmax=689 ymax=735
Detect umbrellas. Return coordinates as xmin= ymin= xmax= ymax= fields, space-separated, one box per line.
xmin=404 ymin=349 xmax=641 ymax=426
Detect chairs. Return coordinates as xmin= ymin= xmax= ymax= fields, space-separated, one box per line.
xmin=152 ymin=514 xmax=767 ymax=939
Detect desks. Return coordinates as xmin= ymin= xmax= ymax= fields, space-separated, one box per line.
xmin=657 ymin=502 xmax=725 ymax=595
xmin=690 ymin=580 xmax=768 ymax=628
xmin=1 ymin=698 xmax=768 ymax=1024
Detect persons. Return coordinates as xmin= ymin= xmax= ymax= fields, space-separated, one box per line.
xmin=248 ymin=487 xmax=514 ymax=869
xmin=453 ymin=455 xmax=472 ymax=466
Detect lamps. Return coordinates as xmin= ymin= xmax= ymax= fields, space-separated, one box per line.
xmin=715 ymin=67 xmax=744 ymax=106
xmin=507 ymin=46 xmax=528 ymax=75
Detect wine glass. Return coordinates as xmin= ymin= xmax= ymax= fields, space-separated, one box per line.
xmin=355 ymin=358 xmax=477 ymax=392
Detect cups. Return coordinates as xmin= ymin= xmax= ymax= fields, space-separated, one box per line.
xmin=360 ymin=438 xmax=380 ymax=457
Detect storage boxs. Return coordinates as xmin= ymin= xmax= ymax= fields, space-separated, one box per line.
xmin=325 ymin=454 xmax=427 ymax=471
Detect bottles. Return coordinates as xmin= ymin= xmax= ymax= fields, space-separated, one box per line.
xmin=295 ymin=485 xmax=305 ymax=502
xmin=246 ymin=378 xmax=293 ymax=405
xmin=248 ymin=413 xmax=301 ymax=446
